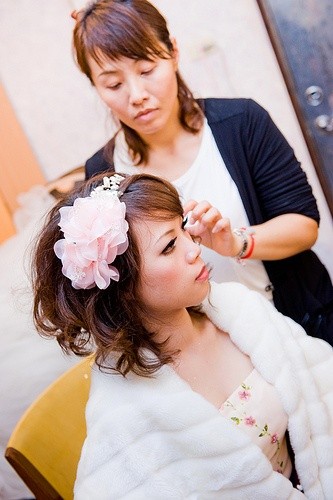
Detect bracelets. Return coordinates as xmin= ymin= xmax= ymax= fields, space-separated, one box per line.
xmin=234 ymin=227 xmax=257 ymax=264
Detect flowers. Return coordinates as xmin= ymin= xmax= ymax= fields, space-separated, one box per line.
xmin=53 ymin=173 xmax=130 ymax=289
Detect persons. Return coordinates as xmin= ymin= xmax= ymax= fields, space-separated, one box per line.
xmin=72 ymin=0 xmax=333 ymax=346
xmin=23 ymin=173 xmax=333 ymax=500
